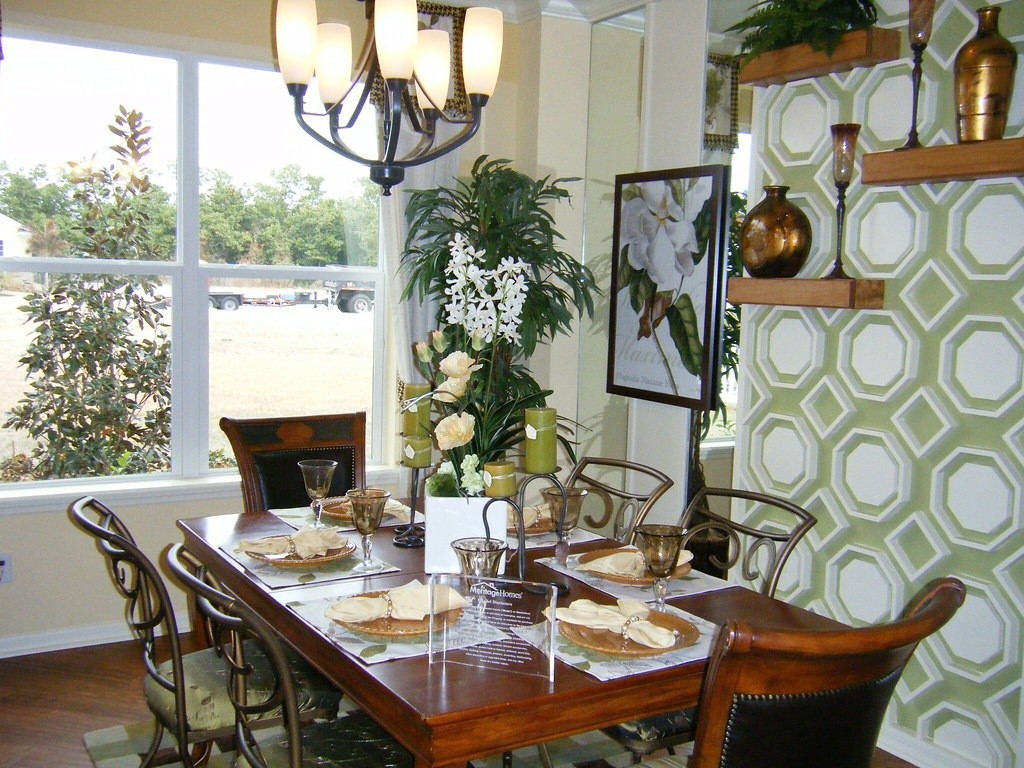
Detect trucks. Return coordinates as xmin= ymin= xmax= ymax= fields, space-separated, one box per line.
xmin=322 ymin=264 xmax=377 ymax=314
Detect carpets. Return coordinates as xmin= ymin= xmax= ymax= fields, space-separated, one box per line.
xmin=83 ymin=693 xmax=633 ymax=768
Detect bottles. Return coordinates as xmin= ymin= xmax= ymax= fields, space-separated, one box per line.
xmin=953 ymin=7 xmax=1017 ymax=143
xmin=742 ymin=184 xmax=813 ymax=278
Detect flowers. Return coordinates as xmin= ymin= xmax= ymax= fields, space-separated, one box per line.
xmin=401 ymin=232 xmax=592 ymax=495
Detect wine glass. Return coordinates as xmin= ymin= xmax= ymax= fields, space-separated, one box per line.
xmin=452 ymin=537 xmax=508 ymax=638
xmin=297 ymin=459 xmax=338 ymax=527
xmin=346 ymin=489 xmax=391 ymax=571
xmin=541 ymin=487 xmax=588 ymax=568
xmin=632 ymin=524 xmax=690 ymax=612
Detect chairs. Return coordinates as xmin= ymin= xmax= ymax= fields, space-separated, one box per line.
xmin=628 ymin=577 xmax=967 ymax=768
xmin=219 ymin=411 xmax=366 ymax=513
xmin=67 ymin=495 xmax=415 ymax=768
xmin=564 ymin=455 xmax=817 ymax=763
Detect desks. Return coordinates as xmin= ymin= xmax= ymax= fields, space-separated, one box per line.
xmin=176 ymin=496 xmax=870 ymax=768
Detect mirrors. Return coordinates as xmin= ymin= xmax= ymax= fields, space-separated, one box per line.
xmin=576 ymin=3 xmax=647 ymax=539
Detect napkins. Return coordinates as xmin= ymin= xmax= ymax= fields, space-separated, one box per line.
xmin=323 ymin=578 xmax=472 ymax=624
xmin=543 ymin=597 xmax=675 ymax=650
xmin=330 ymin=498 xmax=410 ymax=522
xmin=507 ymin=503 xmax=551 ymax=528
xmin=572 ymin=549 xmax=695 ymax=578
xmin=239 ymin=525 xmax=349 ymax=560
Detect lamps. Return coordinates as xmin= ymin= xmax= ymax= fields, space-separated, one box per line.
xmin=276 ymin=0 xmax=503 ymax=197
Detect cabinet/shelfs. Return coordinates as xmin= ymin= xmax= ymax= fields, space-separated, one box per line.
xmin=728 ymin=25 xmax=1024 ymax=310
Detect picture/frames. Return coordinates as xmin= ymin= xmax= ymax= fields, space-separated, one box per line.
xmin=606 ymin=160 xmax=734 ymax=411
xmin=704 ymin=52 xmax=740 ymax=154
xmin=369 ymin=0 xmax=470 ymax=117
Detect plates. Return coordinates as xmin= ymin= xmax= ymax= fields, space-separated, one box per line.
xmin=319 ymin=497 xmax=405 ymax=521
xmin=558 ymin=608 xmax=700 ymax=660
xmin=578 ymin=548 xmax=692 ymax=584
xmin=245 ymin=534 xmax=355 ymax=568
xmin=506 ymin=517 xmax=555 ymax=538
xmin=332 ymin=591 xmax=462 ymax=636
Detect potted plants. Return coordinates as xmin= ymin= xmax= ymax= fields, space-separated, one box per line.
xmin=682 ymin=191 xmax=747 ymax=581
xmin=394 ymin=153 xmax=569 ymax=506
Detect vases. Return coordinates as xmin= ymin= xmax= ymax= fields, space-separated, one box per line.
xmin=738 ymin=185 xmax=812 ymax=278
xmin=425 ymin=497 xmax=506 ymax=580
xmin=953 ymin=5 xmax=1018 ymax=141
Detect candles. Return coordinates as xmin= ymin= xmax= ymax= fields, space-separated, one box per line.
xmin=401 ymin=384 xmax=431 ymax=436
xmin=525 ymin=408 xmax=557 ymax=474
xmin=400 ymin=436 xmax=431 ymax=468
xmin=484 ymin=461 xmax=516 ymax=496
